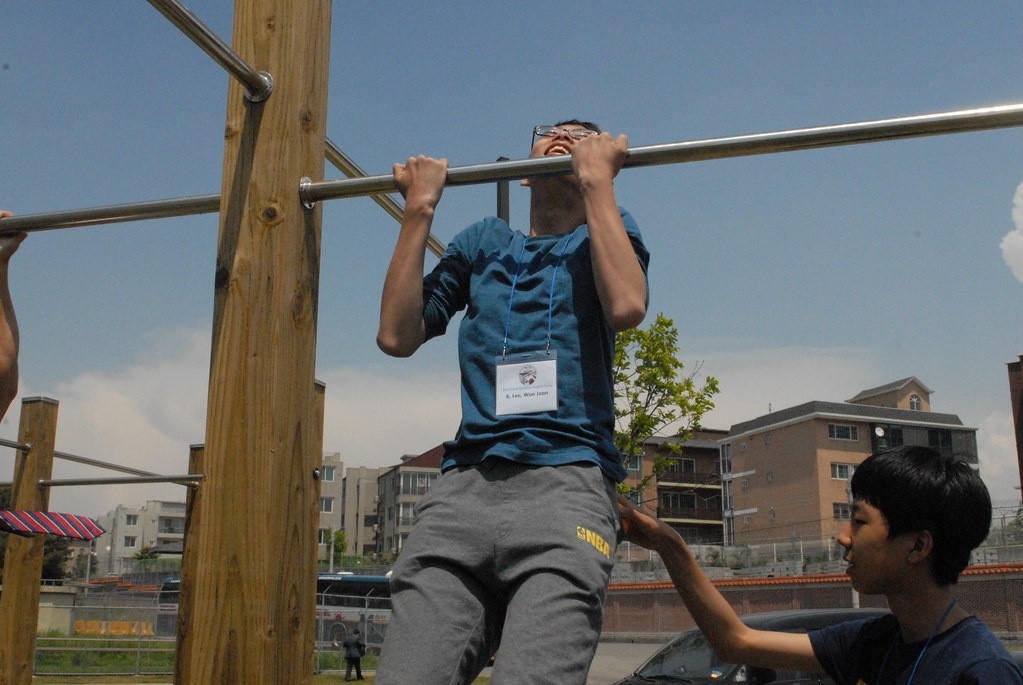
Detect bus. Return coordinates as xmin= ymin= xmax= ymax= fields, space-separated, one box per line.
xmin=156 ymin=570 xmax=391 ymax=656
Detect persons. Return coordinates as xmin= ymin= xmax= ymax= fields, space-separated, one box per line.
xmin=343 ymin=629 xmax=366 ymax=681
xmin=617 ymin=446 xmax=1023 ymax=685
xmin=0 ymin=211 xmax=28 ymax=423
xmin=377 ymin=120 xmax=649 ymax=685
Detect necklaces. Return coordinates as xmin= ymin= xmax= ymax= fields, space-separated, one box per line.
xmin=876 ymin=600 xmax=956 ymax=685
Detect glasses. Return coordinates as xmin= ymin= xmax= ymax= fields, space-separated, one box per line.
xmin=531 ymin=125 xmax=599 ymax=141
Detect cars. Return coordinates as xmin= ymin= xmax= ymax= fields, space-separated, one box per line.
xmin=610 ymin=606 xmax=892 ymax=685
